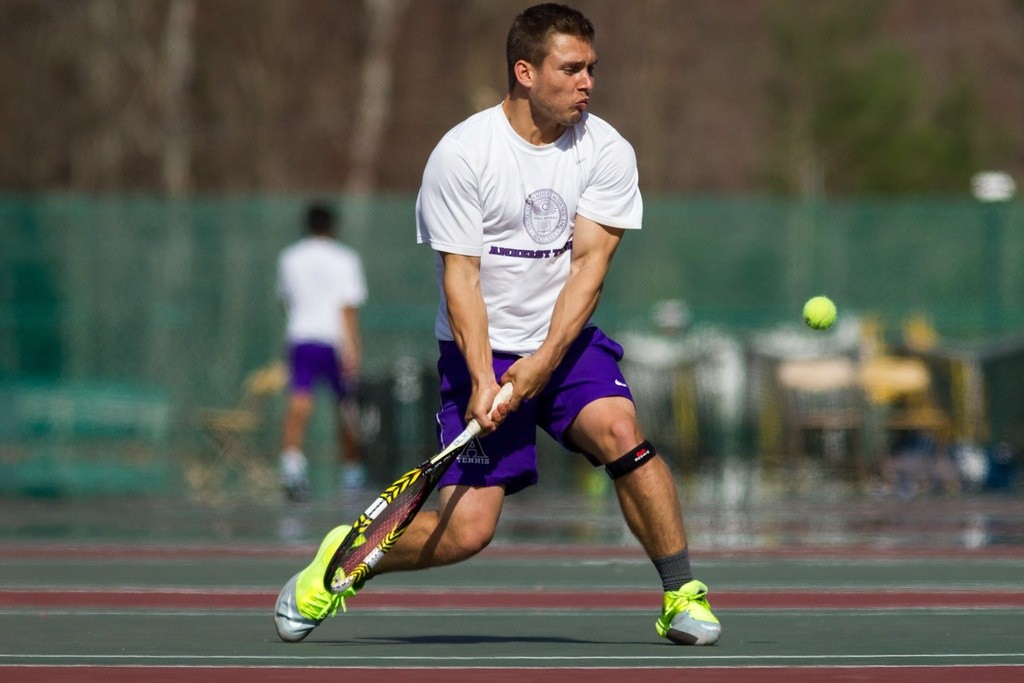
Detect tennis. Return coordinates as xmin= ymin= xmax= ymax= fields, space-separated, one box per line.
xmin=803 ymin=295 xmax=837 ymax=330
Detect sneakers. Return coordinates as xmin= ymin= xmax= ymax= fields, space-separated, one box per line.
xmin=654 ymin=578 xmax=723 ymax=646
xmin=273 ymin=524 xmax=376 ymax=643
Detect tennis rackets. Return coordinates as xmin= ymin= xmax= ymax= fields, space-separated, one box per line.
xmin=322 ymin=383 xmax=514 ymax=595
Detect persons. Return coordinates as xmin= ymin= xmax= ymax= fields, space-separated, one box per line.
xmin=274 ymin=8 xmax=722 ymax=646
xmin=274 ymin=196 xmax=367 ymax=503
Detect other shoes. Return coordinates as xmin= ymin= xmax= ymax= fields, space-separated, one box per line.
xmin=342 ymin=483 xmax=368 ymax=504
xmin=277 ymin=468 xmax=310 ymax=505
